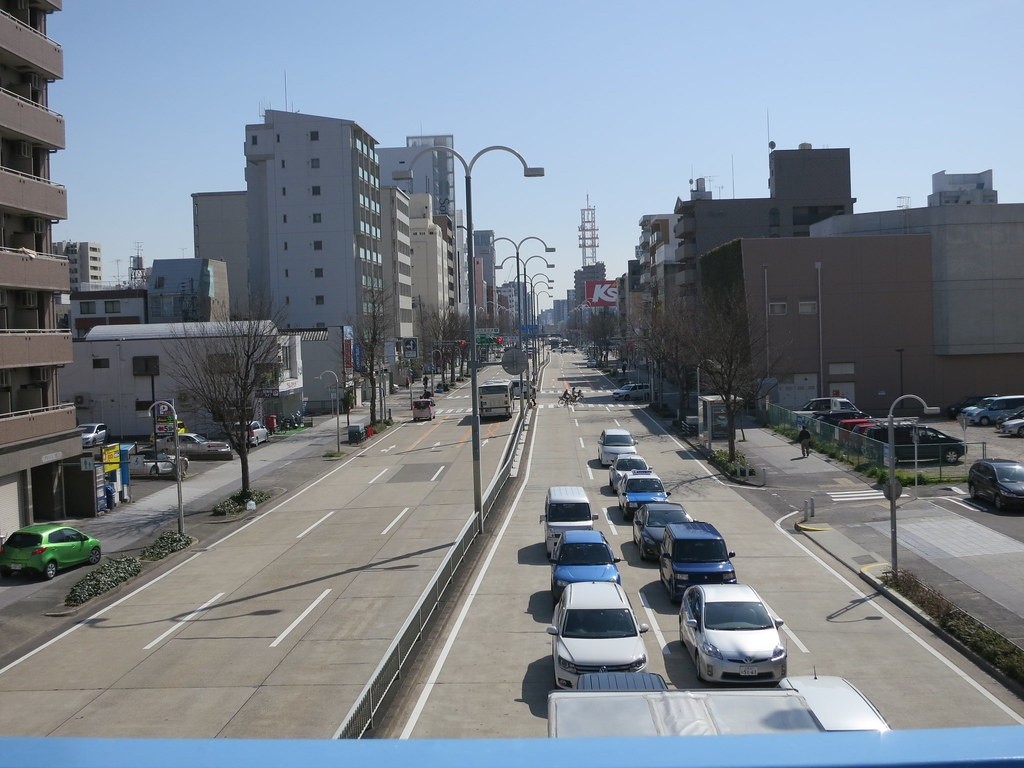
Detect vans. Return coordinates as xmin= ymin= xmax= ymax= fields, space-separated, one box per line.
xmin=412 ymin=399 xmax=436 ymax=421
xmin=510 ymin=379 xmax=534 ymax=398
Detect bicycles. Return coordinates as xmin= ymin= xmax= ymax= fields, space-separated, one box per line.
xmin=557 ymin=395 xmax=585 ymax=408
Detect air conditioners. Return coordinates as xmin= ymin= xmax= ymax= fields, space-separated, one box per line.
xmin=31 ymin=367 xmax=52 ymax=382
xmin=15 ymin=141 xmax=31 ymax=158
xmin=15 ymin=291 xmax=37 ymax=310
xmin=27 ymin=72 xmax=41 ymax=92
xmin=27 ymin=218 xmax=47 ymax=233
xmin=17 ymin=0 xmax=29 ymax=10
xmin=72 ymin=393 xmax=91 ymax=407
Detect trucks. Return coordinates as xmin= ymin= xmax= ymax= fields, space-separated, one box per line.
xmin=546 ymin=688 xmax=826 ymax=740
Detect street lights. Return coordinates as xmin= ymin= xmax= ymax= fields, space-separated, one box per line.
xmin=697 ymin=359 xmax=722 ymax=445
xmin=478 ymin=236 xmax=556 ymax=388
xmin=895 ymin=348 xmax=904 ymax=408
xmin=887 ymin=394 xmax=940 ymax=580
xmin=147 ymin=401 xmax=185 ymax=536
xmin=315 ymin=370 xmax=341 ymax=452
xmin=392 ymin=144 xmax=546 ymax=533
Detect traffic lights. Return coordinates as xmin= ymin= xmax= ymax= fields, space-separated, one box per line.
xmin=488 ymin=337 xmax=503 ymax=343
xmin=456 ymin=340 xmax=465 ymax=345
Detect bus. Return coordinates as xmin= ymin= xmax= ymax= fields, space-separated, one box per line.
xmin=551 ymin=339 xmax=568 ymax=349
xmin=478 ymin=379 xmax=514 ymax=419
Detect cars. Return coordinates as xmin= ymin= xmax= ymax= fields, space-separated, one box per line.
xmin=539 ymin=428 xmax=894 ymax=732
xmin=587 ymin=359 xmax=597 ymax=368
xmin=552 ymin=345 xmax=576 ymax=352
xmin=76 ymin=418 xmax=270 ymax=481
xmin=968 ymin=457 xmax=1024 ymax=508
xmin=0 ymin=522 xmax=102 ymax=580
xmin=612 ymin=383 xmax=650 ymax=402
xmin=787 ymin=394 xmax=1024 ymax=466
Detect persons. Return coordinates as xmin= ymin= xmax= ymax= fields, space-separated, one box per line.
xmin=532 ymin=389 xmax=536 ymax=406
xmin=622 ymin=365 xmax=626 ymax=374
xmin=423 ymin=376 xmax=428 ymax=389
xmin=799 ymin=425 xmax=811 ymax=457
xmin=563 ymin=387 xmax=581 ymax=405
xmin=406 ymin=376 xmax=410 ymax=390
xmin=422 ymin=390 xmax=431 ymax=399
